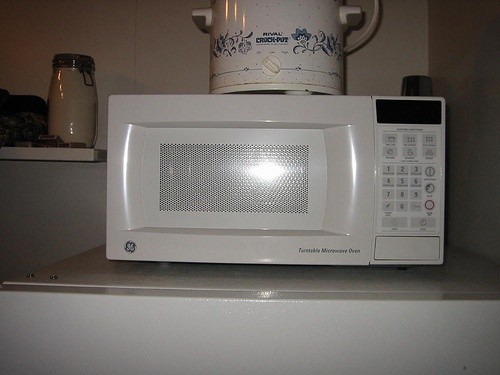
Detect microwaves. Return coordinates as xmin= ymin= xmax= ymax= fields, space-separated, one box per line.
xmin=108 ymin=93 xmax=446 ymax=267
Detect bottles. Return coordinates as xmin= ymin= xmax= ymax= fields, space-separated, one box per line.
xmin=48 ymin=53 xmax=98 ymax=148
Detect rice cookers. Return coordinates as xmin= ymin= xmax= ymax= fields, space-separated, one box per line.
xmin=192 ymin=0 xmax=380 ymax=95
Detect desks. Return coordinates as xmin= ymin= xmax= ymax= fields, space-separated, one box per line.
xmin=1 ymin=242 xmax=500 ymax=374
